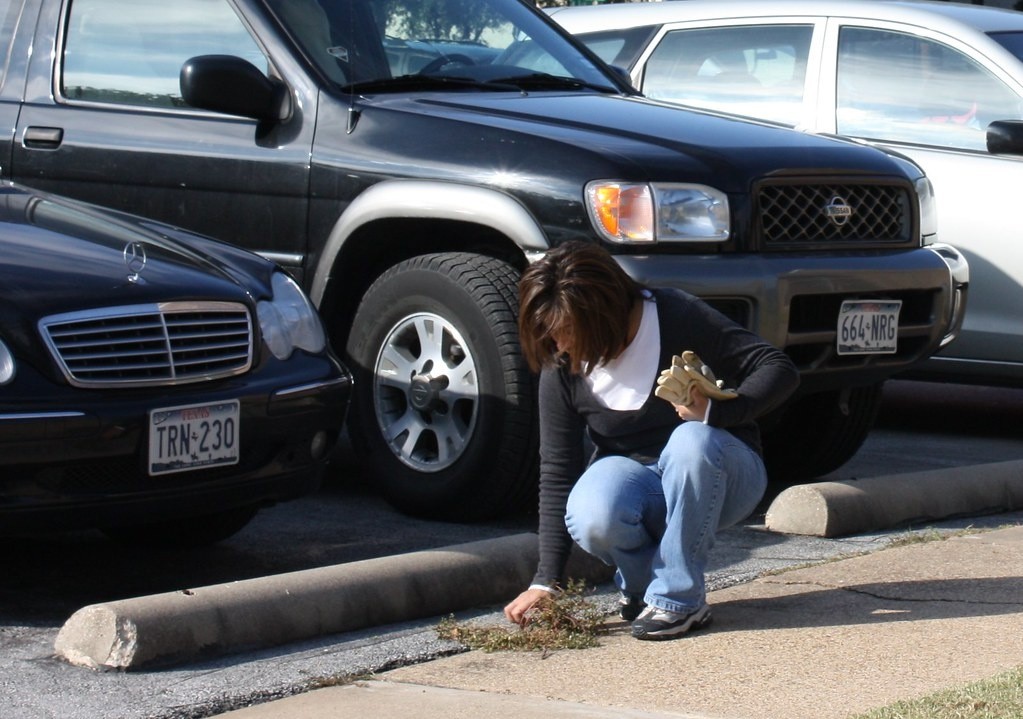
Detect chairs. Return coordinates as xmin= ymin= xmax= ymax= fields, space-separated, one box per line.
xmin=278 ymin=0 xmax=347 ymax=87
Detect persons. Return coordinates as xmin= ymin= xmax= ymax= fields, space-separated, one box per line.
xmin=504 ymin=243 xmax=799 ymax=640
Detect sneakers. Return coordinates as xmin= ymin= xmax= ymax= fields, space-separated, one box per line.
xmin=619 ymin=596 xmax=647 ymax=620
xmin=631 ymin=602 xmax=714 ymax=640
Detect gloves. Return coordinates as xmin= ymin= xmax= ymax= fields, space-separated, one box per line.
xmin=654 ymin=351 xmax=739 ymax=407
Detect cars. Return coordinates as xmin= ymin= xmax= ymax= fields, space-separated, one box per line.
xmin=1 ymin=176 xmax=357 ymax=553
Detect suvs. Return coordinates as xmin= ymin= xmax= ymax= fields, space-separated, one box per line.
xmin=465 ymin=0 xmax=1023 ymax=395
xmin=1 ymin=0 xmax=972 ymax=523
